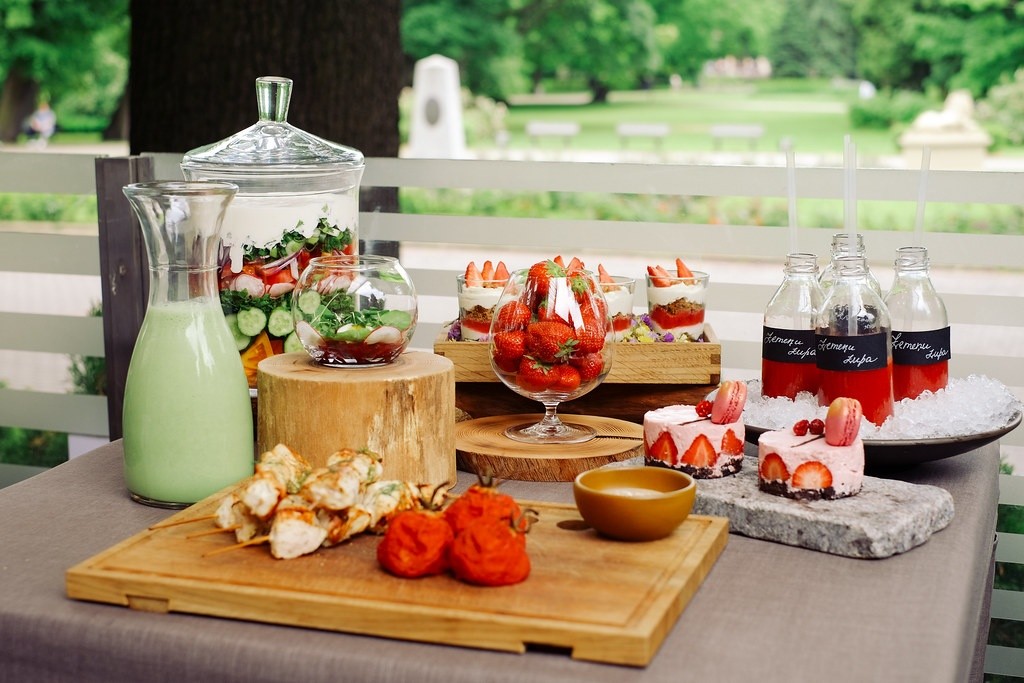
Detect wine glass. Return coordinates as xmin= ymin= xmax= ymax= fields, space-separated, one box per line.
xmin=487 ymin=267 xmax=616 ymax=445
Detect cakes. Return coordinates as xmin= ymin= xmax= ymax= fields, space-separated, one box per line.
xmin=642 ymin=380 xmax=748 ymax=478
xmin=758 ymin=398 xmax=865 ymax=501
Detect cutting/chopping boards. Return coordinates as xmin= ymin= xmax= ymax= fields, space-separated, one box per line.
xmin=64 ymin=468 xmax=732 ymax=666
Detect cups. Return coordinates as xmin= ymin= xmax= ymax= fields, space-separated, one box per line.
xmin=587 ymin=275 xmax=637 ymax=343
xmin=457 ymin=271 xmax=527 ymax=345
xmin=644 ymin=267 xmax=711 ymax=340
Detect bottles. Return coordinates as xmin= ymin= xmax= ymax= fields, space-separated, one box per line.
xmin=181 ymin=71 xmax=367 ymax=408
xmin=293 ymin=251 xmax=416 ymax=369
xmin=761 ymin=252 xmax=824 ymax=399
xmin=815 ymin=258 xmax=895 ymax=429
xmin=884 ymin=246 xmax=951 ymax=401
xmin=815 ymin=234 xmax=882 ymax=311
xmin=120 ymin=182 xmax=256 ymax=512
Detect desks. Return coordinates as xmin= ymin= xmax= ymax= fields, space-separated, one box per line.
xmin=0 ymin=437 xmax=1000 ymax=683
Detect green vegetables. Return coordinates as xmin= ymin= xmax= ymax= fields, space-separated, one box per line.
xmin=219 ymin=287 xmax=390 ymax=341
xmin=244 ymin=212 xmax=353 ymax=260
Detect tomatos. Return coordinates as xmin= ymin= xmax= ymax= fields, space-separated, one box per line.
xmin=375 ymin=471 xmax=541 ymax=585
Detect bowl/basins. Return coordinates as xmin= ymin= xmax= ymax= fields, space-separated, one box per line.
xmin=574 ymin=462 xmax=696 ymax=542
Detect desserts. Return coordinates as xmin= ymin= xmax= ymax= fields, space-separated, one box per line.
xmin=458 ymin=259 xmax=705 ymax=342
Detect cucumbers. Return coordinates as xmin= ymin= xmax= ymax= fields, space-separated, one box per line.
xmin=380 ymin=310 xmax=411 ymax=331
xmin=223 ymin=291 xmax=320 ymax=355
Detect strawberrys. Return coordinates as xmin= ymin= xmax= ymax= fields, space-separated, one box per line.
xmin=492 ymin=260 xmax=609 ymax=392
